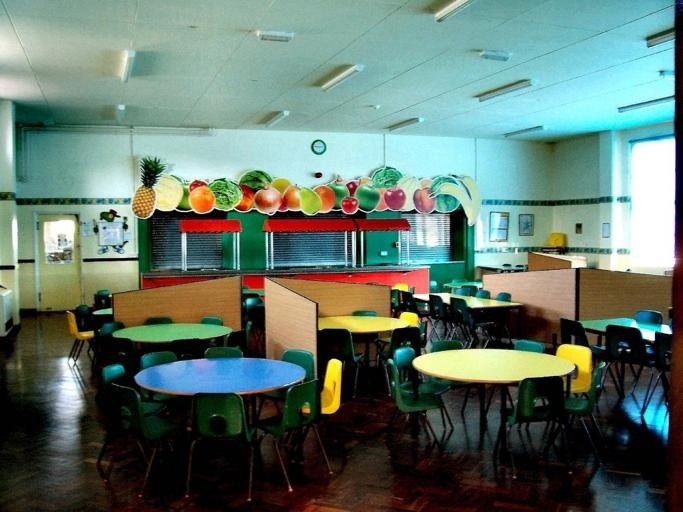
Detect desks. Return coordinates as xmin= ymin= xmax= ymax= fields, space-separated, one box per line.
xmin=542 ymin=247 xmax=569 ymax=255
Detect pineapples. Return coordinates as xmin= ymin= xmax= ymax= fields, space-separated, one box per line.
xmin=132 ymin=155 xmax=167 ymax=219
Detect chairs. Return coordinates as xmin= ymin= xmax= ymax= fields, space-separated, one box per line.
xmin=204 ymin=347 xmax=243 ymax=358
xmin=97 ymin=364 xmax=161 ymax=467
xmin=256 ymin=378 xmax=335 ymax=490
xmin=379 ymin=312 xmax=419 ymax=382
xmin=392 ymin=289 xmax=400 ymax=317
xmin=402 ymin=291 xmax=440 ymax=340
xmin=455 ymin=288 xmax=471 ymax=296
xmin=65 ymin=310 xmax=94 ymax=362
xmin=227 ymin=321 xmax=251 ymax=351
xmin=391 ymin=345 xmax=451 ymax=443
xmin=350 ymin=310 xmax=380 ymax=366
xmin=555 ymin=344 xmax=593 ymax=423
xmin=225 ymin=330 xmax=246 ymax=348
xmin=541 ymin=233 xmax=568 ymax=255
xmin=141 ymin=351 xmax=191 ymax=406
xmin=100 ymin=322 xmax=124 ymax=335
xmin=321 ymin=328 xmax=365 ymax=399
xmin=504 ymin=376 xmax=566 ymax=466
xmin=143 ymin=316 xmax=172 ymax=324
xmin=108 ymin=382 xmax=182 ymax=497
xmin=200 ymin=316 xmax=222 ymax=326
xmin=606 ymin=324 xmax=655 ymax=413
xmin=558 ymin=318 xmax=621 ymax=401
xmin=515 ymin=265 xmax=524 ymax=272
xmin=541 ymin=361 xmax=607 ymax=461
xmin=376 ymin=327 xmax=421 ymax=397
xmin=643 ymin=332 xmax=672 ymax=413
xmin=184 ymin=392 xmax=260 ymax=503
xmin=170 ymin=338 xmax=201 ymax=357
xmin=242 ymin=294 xmax=265 ymax=343
xmin=501 ymin=264 xmax=511 ymax=272
xmin=430 ymin=280 xmax=439 ymax=292
xmin=484 ymin=340 xmax=545 ymax=416
xmin=450 ymin=297 xmax=495 ymax=349
xmin=632 ymin=310 xmax=663 ymax=395
xmin=462 ymin=285 xmax=478 ymax=296
xmin=429 ymin=340 xmax=473 ymax=420
xmin=428 ymin=294 xmax=467 ymax=341
xmin=476 ymin=290 xmax=490 ymax=299
xmin=495 ymin=292 xmax=511 ymax=302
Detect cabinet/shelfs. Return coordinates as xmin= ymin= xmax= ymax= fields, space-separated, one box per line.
xmin=140 ymin=266 xmax=430 ymax=300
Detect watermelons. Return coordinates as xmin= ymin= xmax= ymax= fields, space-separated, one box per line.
xmin=239 ymin=170 xmax=272 ymax=191
xmin=431 ymin=176 xmax=461 ymax=212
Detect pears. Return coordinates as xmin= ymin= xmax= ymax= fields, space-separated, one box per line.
xmin=271 ymin=178 xmax=289 ymax=192
xmin=299 ymin=184 xmax=322 ymax=215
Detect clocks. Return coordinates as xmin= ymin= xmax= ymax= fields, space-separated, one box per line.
xmin=311 ymin=139 xmax=325 ymax=155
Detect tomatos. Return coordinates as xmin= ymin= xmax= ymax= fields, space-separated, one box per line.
xmin=189 ymin=179 xmax=207 ymax=192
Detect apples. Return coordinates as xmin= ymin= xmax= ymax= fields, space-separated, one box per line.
xmin=354 ymin=184 xmax=381 ymax=212
xmin=234 ymin=184 xmax=255 ymax=211
xmin=341 ymin=196 xmax=358 ymax=213
xmin=327 ymin=181 xmax=350 ymax=210
xmin=384 ymin=186 xmax=406 ymax=210
xmin=346 ymin=182 xmax=358 ymax=196
xmin=176 ymin=180 xmax=192 ymax=211
xmin=282 ymin=184 xmax=301 ymax=210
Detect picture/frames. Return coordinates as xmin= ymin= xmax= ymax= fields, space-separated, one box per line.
xmin=519 ymin=214 xmax=535 ymax=236
xmin=489 ymin=212 xmax=509 ymax=243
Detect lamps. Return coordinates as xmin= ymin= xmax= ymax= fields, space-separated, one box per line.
xmin=266 ymin=111 xmax=289 ymax=129
xmin=480 ymin=50 xmax=510 ymax=62
xmin=120 ymin=52 xmax=134 ymax=83
xmin=320 ymin=63 xmax=363 ymax=92
xmin=388 ymin=117 xmax=423 ymax=131
xmin=435 ymin=0 xmax=472 ymax=23
xmin=477 ymin=80 xmax=533 ymax=104
xmin=645 ymin=26 xmax=675 ymax=48
xmin=503 ymin=124 xmax=543 ymax=137
xmin=617 ymin=94 xmax=674 ymax=115
xmin=258 ymin=30 xmax=294 ymax=43
xmin=117 ymin=104 xmax=124 ymax=120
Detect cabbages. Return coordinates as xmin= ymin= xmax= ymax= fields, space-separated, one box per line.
xmin=396 ymin=173 xmax=421 ymax=211
xmin=372 ymin=167 xmax=403 ymax=191
xmin=206 ymin=179 xmax=243 ymax=211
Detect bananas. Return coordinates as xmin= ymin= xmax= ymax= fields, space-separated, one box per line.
xmin=462 ymin=176 xmax=480 ymax=226
xmin=428 ymin=183 xmax=472 ymax=218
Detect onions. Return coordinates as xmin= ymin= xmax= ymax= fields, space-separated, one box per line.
xmin=253 ymin=185 xmax=281 ymax=214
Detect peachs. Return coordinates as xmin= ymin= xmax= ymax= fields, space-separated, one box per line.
xmin=414 ymin=188 xmax=435 ymax=213
